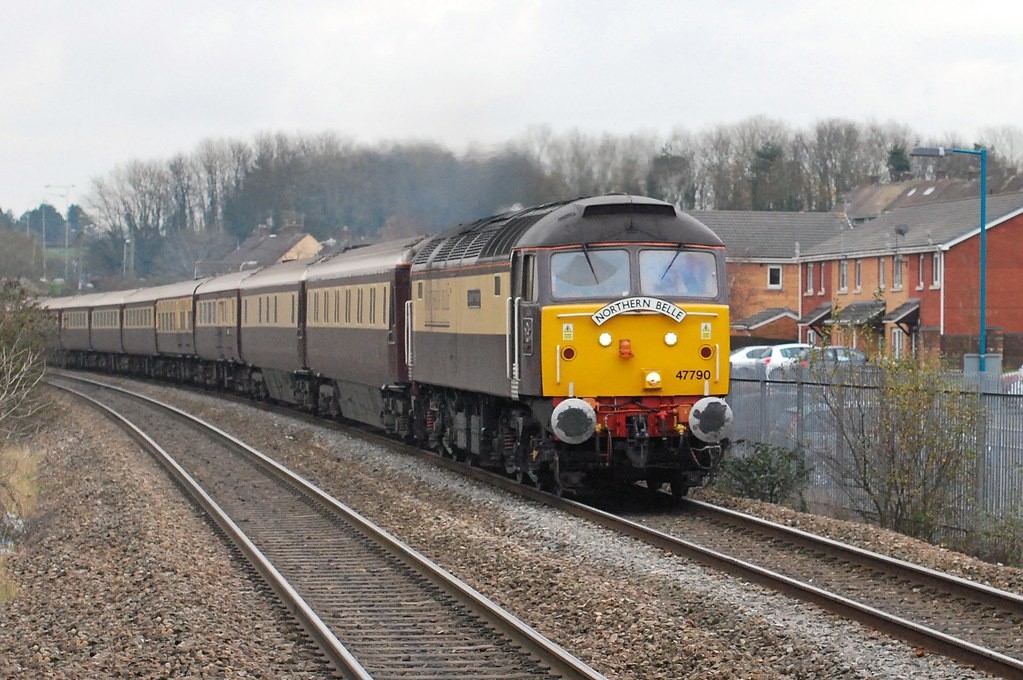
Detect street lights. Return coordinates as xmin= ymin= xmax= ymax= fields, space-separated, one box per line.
xmin=908 ymin=147 xmax=989 ymax=373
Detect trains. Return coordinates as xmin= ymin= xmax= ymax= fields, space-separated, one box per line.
xmin=0 ymin=191 xmax=736 ymax=518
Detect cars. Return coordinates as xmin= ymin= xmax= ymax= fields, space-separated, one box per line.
xmin=728 ymin=345 xmax=791 ymax=379
xmin=791 ymin=346 xmax=891 ymax=372
xmin=756 ymin=343 xmax=849 ymax=388
